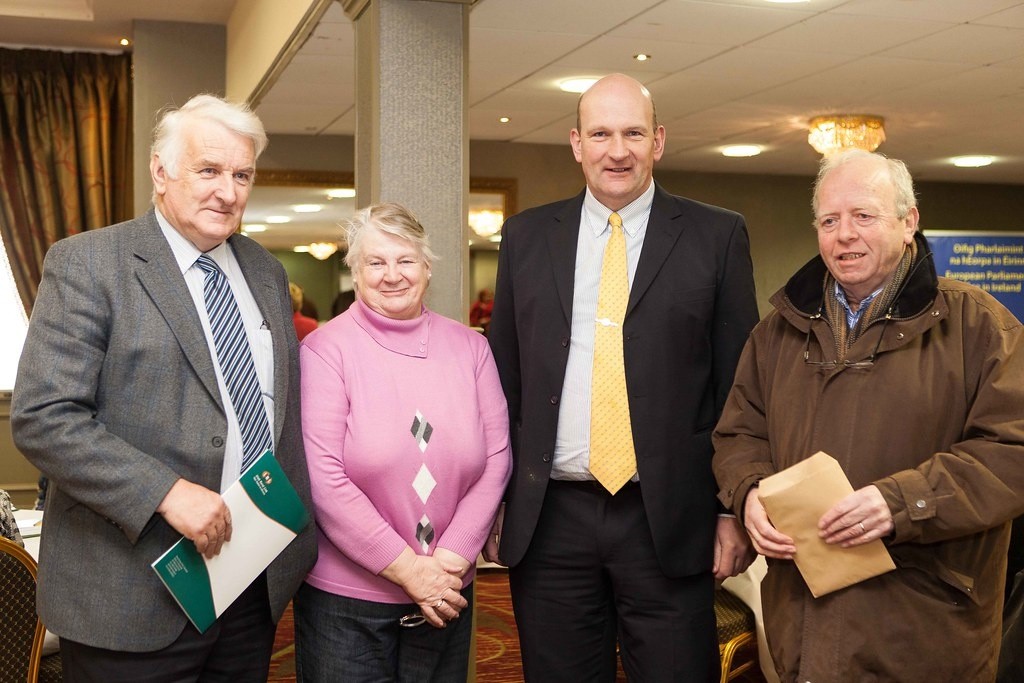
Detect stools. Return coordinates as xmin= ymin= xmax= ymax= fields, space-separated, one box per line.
xmin=713 ymin=549 xmax=763 ymax=683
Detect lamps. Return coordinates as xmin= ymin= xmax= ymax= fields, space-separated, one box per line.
xmin=308 ymin=243 xmax=337 ymax=260
xmin=807 ymin=114 xmax=886 ymax=157
xmin=468 ymin=209 xmax=502 ymax=237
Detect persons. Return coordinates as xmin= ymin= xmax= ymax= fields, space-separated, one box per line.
xmin=288 ymin=281 xmax=317 ymax=341
xmin=0 ymin=489 xmax=25 ymax=548
xmin=10 ymin=92 xmax=312 ymax=683
xmin=482 ymin=72 xmax=761 ymax=683
xmin=712 ymin=150 xmax=1024 ymax=683
xmin=471 ymin=289 xmax=494 ymax=338
xmin=292 ymin=203 xmax=514 ymax=682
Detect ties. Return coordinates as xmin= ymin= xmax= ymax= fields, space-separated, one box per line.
xmin=196 ymin=253 xmax=273 ymax=476
xmin=588 ymin=212 xmax=636 ymax=497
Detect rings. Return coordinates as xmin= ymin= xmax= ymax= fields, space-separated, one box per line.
xmin=859 ymin=523 xmax=866 ymax=532
xmin=436 ymin=600 xmax=443 ymax=608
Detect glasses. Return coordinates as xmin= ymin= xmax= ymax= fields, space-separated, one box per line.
xmin=804 ymin=301 xmax=899 ymax=370
xmin=399 ymin=612 xmax=427 ymax=627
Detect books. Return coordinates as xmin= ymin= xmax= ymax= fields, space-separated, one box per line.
xmin=151 ymin=448 xmax=310 ymax=635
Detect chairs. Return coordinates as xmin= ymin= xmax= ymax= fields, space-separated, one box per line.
xmin=0 ymin=533 xmax=46 ymax=683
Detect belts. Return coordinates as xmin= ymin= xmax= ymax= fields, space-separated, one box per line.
xmin=559 ymin=481 xmax=639 ymax=494
